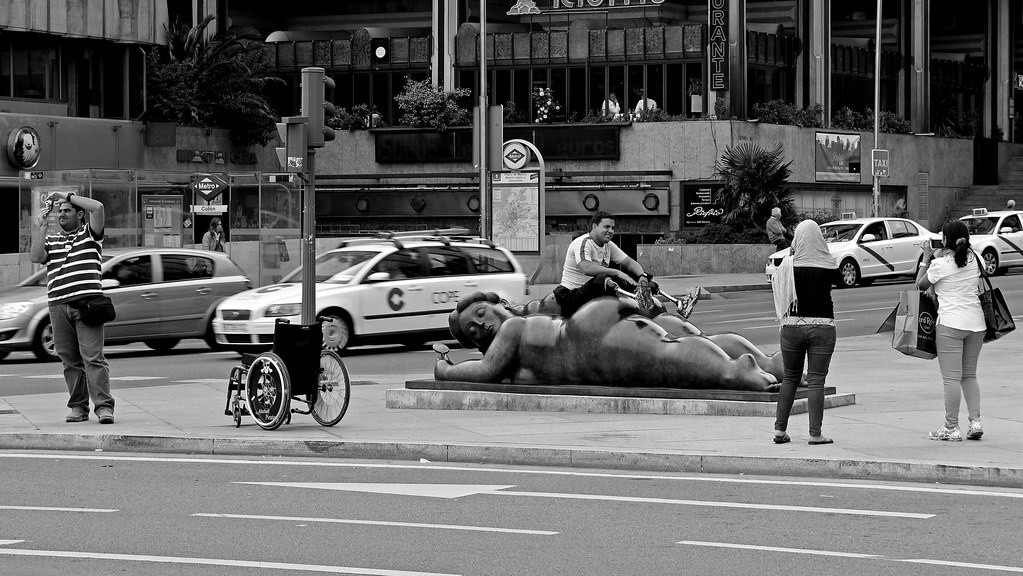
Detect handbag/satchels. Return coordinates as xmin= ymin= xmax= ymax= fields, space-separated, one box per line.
xmin=876 ymin=282 xmax=938 ymax=360
xmin=66 ymin=295 xmax=115 ymax=327
xmin=970 ymin=247 xmax=1016 ymax=343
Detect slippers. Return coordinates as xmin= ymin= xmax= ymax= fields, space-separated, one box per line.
xmin=773 ymin=431 xmax=791 ymax=443
xmin=808 ymin=435 xmax=833 ymax=445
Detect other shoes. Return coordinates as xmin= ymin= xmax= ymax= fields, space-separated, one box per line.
xmin=66 ymin=407 xmax=90 ymax=422
xmin=99 ymin=409 xmax=115 ymax=424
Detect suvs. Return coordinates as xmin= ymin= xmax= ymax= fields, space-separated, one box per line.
xmin=211 ymin=226 xmax=529 ymax=359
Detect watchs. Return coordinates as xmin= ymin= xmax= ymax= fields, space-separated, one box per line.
xmin=639 ymin=273 xmax=648 ymax=277
xmin=919 ymin=263 xmax=929 ymax=268
xmin=67 ymin=192 xmax=75 ymax=202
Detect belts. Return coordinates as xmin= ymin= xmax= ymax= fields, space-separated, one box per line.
xmin=781 ymin=316 xmax=836 ymax=326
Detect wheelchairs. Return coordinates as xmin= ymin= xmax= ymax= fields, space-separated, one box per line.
xmin=225 ymin=318 xmax=352 ymax=430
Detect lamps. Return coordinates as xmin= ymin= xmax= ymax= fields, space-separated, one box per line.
xmin=192 ymin=150 xmax=224 ymax=165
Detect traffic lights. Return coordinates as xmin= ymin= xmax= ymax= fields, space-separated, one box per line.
xmin=275 ymin=118 xmax=307 ymax=173
xmin=302 ymin=67 xmax=337 ymax=146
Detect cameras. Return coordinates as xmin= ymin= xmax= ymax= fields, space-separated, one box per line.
xmin=45 ymin=199 xmax=54 ymax=210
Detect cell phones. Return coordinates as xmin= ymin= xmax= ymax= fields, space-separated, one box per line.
xmin=932 ymin=240 xmax=944 ymax=249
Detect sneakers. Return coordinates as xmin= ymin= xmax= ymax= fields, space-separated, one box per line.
xmin=966 ymin=418 xmax=984 ymax=440
xmin=929 ymin=422 xmax=962 ymax=441
xmin=676 ymin=285 xmax=701 ymax=319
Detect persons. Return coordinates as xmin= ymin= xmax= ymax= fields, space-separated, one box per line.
xmin=1003 ymin=200 xmax=1016 ymax=211
xmin=772 ymin=219 xmax=844 ymax=445
xmin=435 ymin=293 xmax=809 ymax=390
xmin=601 ymin=92 xmax=620 ymax=119
xmin=554 ymin=211 xmax=700 ymax=317
xmin=635 ymin=90 xmax=657 ymax=118
xmin=29 ymin=191 xmax=116 ymax=424
xmin=202 ymin=217 xmax=225 ymax=252
xmin=765 ymin=207 xmax=791 ymax=251
xmin=916 ymin=220 xmax=988 ymax=442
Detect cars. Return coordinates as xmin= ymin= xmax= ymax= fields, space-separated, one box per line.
xmin=0 ymin=247 xmax=253 ymax=363
xmin=766 ymin=211 xmax=945 ymax=288
xmin=937 ymin=209 xmax=1023 ymax=276
